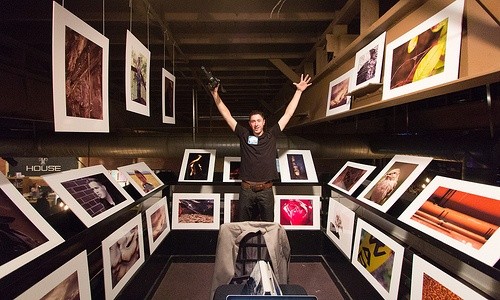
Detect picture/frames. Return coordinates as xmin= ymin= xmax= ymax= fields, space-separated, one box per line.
xmin=0 ymin=0 xmax=500 ymax=300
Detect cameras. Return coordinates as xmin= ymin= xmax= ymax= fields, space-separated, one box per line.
xmin=201 ymin=66 xmax=219 ymax=91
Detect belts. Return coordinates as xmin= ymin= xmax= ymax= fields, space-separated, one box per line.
xmin=241 ymin=182 xmax=273 ymax=191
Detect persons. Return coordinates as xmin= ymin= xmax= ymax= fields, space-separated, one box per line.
xmin=135 ymin=170 xmax=155 ymax=193
xmin=87 ymin=177 xmax=127 ymax=210
xmin=209 ymin=73 xmax=313 ymax=223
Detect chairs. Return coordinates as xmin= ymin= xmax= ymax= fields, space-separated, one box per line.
xmin=221 ymin=224 xmax=280 ymax=284
